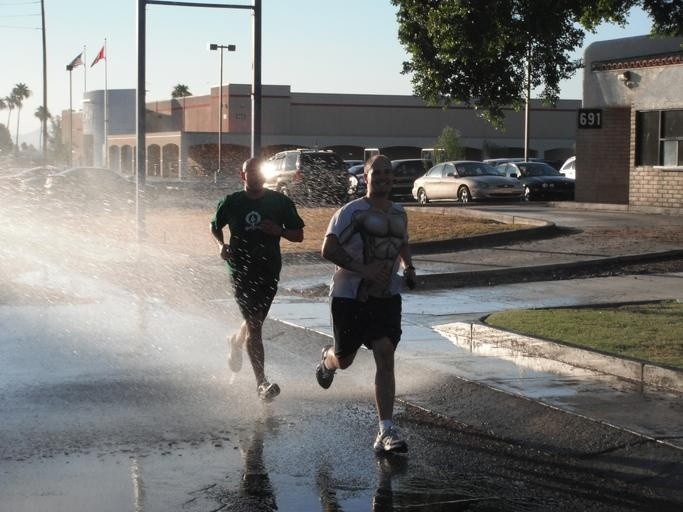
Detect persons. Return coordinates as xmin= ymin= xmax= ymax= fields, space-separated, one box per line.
xmin=313 ymin=154 xmax=420 ymax=456
xmin=312 ymin=455 xmax=410 ymax=512
xmin=206 ymin=157 xmax=306 ymax=404
xmin=228 ymin=405 xmax=279 ymax=511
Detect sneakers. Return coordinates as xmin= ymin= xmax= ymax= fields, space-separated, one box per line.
xmin=315 ymin=344 xmax=337 ymax=390
xmin=373 ymin=427 xmax=409 ymax=454
xmin=226 ymin=333 xmax=244 ymax=373
xmin=257 ymin=381 xmax=280 ymax=404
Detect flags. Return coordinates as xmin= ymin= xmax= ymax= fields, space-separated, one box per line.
xmin=65 ymin=50 xmax=84 ymax=71
xmin=91 ymin=45 xmax=105 ymax=67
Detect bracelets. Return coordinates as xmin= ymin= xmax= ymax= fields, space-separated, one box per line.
xmin=403 ymin=265 xmax=414 ymax=271
xmin=278 ymin=226 xmax=287 ymax=238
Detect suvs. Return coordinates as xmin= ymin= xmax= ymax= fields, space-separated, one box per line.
xmin=258 ymin=146 xmax=353 ymax=207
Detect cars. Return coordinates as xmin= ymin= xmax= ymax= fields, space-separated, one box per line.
xmin=342 ymin=154 xmax=578 ymax=206
xmin=0 ymin=165 xmax=161 ymax=207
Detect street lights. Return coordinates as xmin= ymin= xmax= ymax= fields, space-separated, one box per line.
xmin=208 ymin=42 xmax=236 ymax=185
xmin=65 ymin=64 xmax=73 ymax=166
